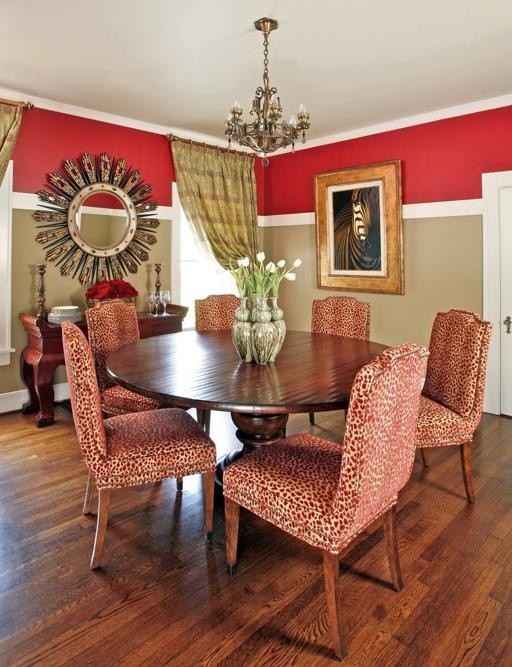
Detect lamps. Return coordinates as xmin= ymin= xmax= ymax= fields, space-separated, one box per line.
xmin=221 ymin=14 xmax=313 ymax=168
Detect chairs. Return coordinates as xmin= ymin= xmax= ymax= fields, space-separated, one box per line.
xmin=190 ymin=292 xmax=243 ymax=338
xmin=82 ymin=295 xmax=212 ymax=439
xmin=55 ymin=316 xmax=226 ymax=580
xmin=307 ymin=294 xmax=371 ymax=430
xmin=219 ymin=339 xmax=438 ymax=660
xmin=404 ymin=304 xmax=495 ymax=515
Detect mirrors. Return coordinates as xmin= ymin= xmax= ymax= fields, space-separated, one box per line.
xmin=27 ymin=146 xmax=168 ymax=289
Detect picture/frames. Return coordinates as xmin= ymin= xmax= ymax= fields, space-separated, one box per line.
xmin=311 ymin=157 xmax=407 ymax=300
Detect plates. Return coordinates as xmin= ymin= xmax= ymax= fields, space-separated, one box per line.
xmin=48 ymin=304 xmax=84 ymax=324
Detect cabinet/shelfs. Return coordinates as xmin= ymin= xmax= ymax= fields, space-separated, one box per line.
xmin=17 ymin=300 xmax=196 ymax=431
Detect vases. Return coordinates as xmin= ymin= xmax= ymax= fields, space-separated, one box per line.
xmin=232 ymin=300 xmax=289 ymax=364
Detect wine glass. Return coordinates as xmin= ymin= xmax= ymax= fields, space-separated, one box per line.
xmin=147 ymin=288 xmax=172 ymax=316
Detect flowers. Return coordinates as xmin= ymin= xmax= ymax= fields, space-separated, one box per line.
xmin=84 ymin=278 xmax=144 ymax=302
xmin=225 ymin=250 xmax=304 ymax=312
